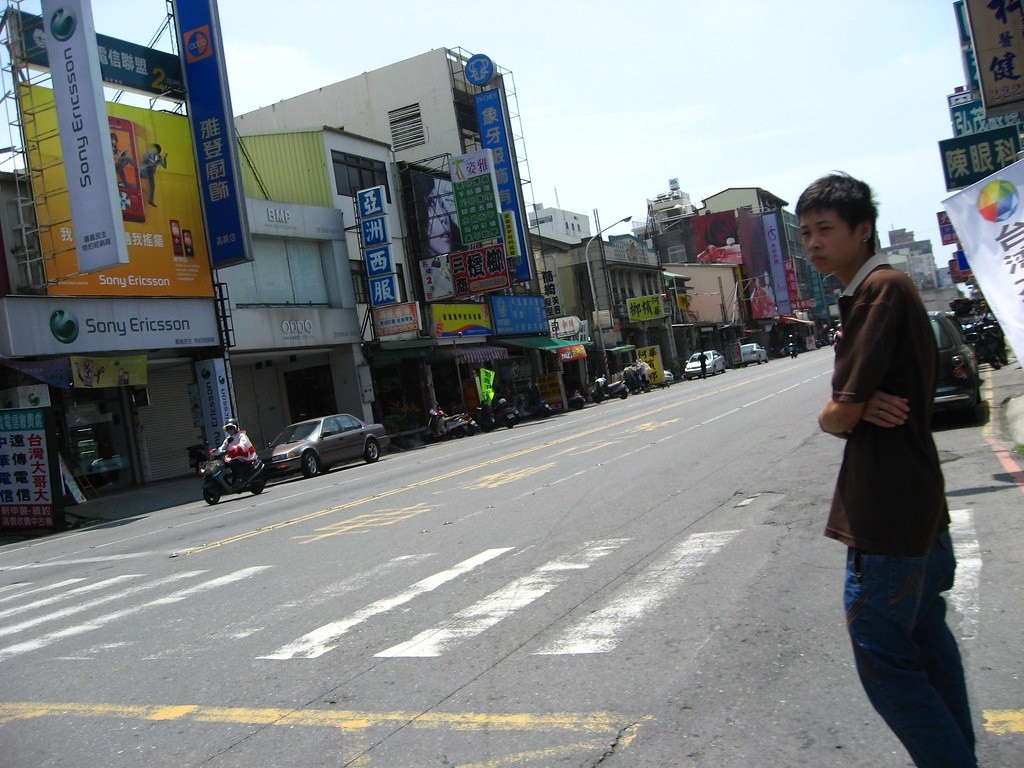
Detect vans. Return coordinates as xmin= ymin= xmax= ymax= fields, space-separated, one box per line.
xmin=926 ymin=310 xmax=985 ymax=420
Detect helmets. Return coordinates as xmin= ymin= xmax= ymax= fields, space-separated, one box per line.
xmin=222 ymin=419 xmax=238 ymax=430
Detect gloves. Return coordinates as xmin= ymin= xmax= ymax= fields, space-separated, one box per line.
xmin=229 ymin=448 xmax=234 ymax=454
xmin=208 ymin=449 xmax=214 ymax=455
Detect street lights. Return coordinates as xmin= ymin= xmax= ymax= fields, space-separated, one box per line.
xmin=585 ymin=215 xmax=633 ymax=381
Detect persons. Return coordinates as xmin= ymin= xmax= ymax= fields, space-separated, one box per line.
xmin=139 ymin=143 xmax=168 ymax=208
xmin=209 ymin=418 xmax=256 ymax=489
xmin=412 ymin=172 xmax=462 ymax=259
xmin=788 ymin=334 xmax=795 ymax=342
xmin=698 ymin=349 xmax=708 ymax=379
xmin=110 ymin=131 xmax=137 ymax=187
xmin=795 ymin=176 xmax=979 ymax=768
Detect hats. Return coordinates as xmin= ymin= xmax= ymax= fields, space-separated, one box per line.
xmin=789 ymin=335 xmax=792 ymax=337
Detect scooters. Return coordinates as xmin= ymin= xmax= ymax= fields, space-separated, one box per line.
xmin=960 ymin=314 xmax=1008 ymax=370
xmin=786 ymin=342 xmax=798 ymax=359
xmin=420 ymin=395 xmax=521 ymax=444
xmin=514 ymin=393 xmax=553 ymax=421
xmin=826 ymin=333 xmax=834 ymax=347
xmin=197 ymin=436 xmax=270 ymax=506
xmin=566 ymin=389 xmax=586 ymax=409
xmin=590 ymin=374 xmax=630 ymax=404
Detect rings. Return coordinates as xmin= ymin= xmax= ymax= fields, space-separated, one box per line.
xmin=876 ymin=409 xmax=883 ymax=418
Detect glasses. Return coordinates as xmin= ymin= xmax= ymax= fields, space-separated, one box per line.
xmin=228 ymin=428 xmax=233 ymax=429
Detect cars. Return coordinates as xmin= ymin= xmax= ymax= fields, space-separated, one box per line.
xmin=663 ymin=370 xmax=675 ymax=385
xmin=257 ymin=413 xmax=391 ymax=478
xmin=684 ymin=350 xmax=726 ymax=380
xmin=735 ymin=343 xmax=769 ymax=368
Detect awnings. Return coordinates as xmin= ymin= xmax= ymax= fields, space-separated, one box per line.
xmin=495 ymin=335 xmax=595 ymax=354
xmin=781 ymin=316 xmax=814 ymax=326
xmin=434 ymin=344 xmax=511 ymax=365
xmin=605 ymin=343 xmax=635 ymax=355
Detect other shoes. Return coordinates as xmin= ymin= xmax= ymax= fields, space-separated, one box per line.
xmin=233 ymin=481 xmax=243 ymax=487
